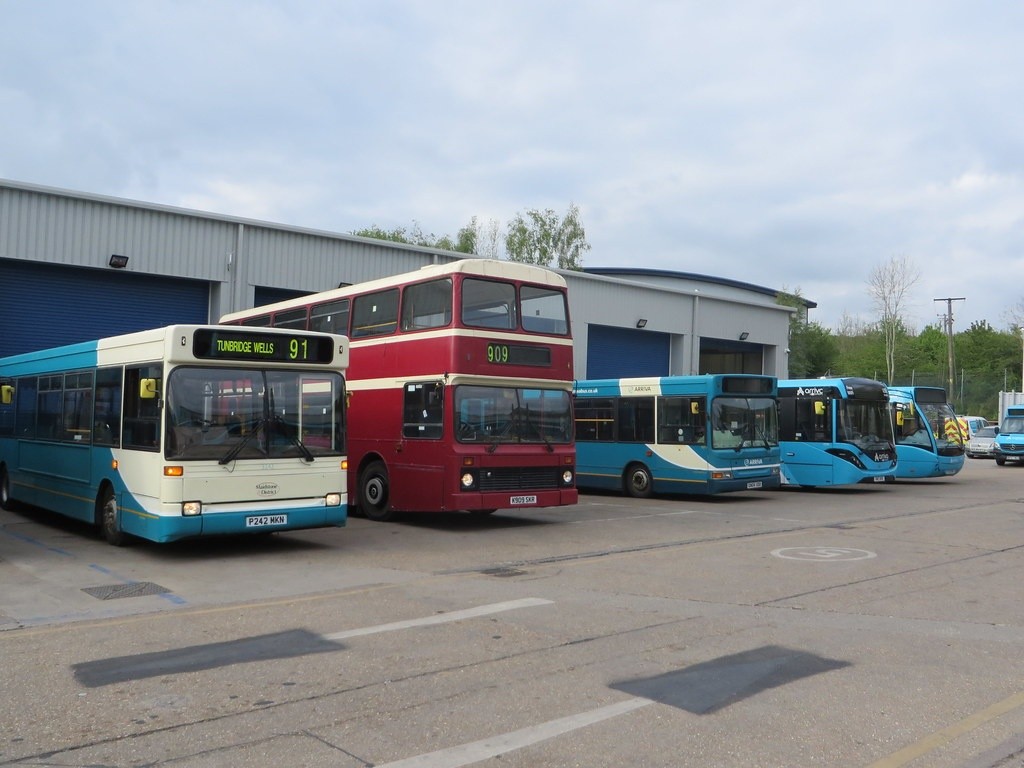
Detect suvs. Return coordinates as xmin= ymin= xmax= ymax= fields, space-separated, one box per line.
xmin=992 ymin=405 xmax=1024 ymax=466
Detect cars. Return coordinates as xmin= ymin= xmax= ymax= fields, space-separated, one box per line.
xmin=965 ymin=426 xmax=999 ymax=459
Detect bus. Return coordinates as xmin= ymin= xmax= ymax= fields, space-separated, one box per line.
xmin=459 ymin=372 xmax=781 ymax=493
xmin=721 ymin=377 xmax=897 ymax=490
xmin=885 ymin=387 xmax=966 ymax=477
xmin=61 ymin=257 xmax=580 ymax=525
xmin=1 ymin=323 xmax=348 ymax=544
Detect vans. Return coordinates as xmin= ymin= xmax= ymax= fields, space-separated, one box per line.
xmin=961 ymin=414 xmax=989 ymax=433
